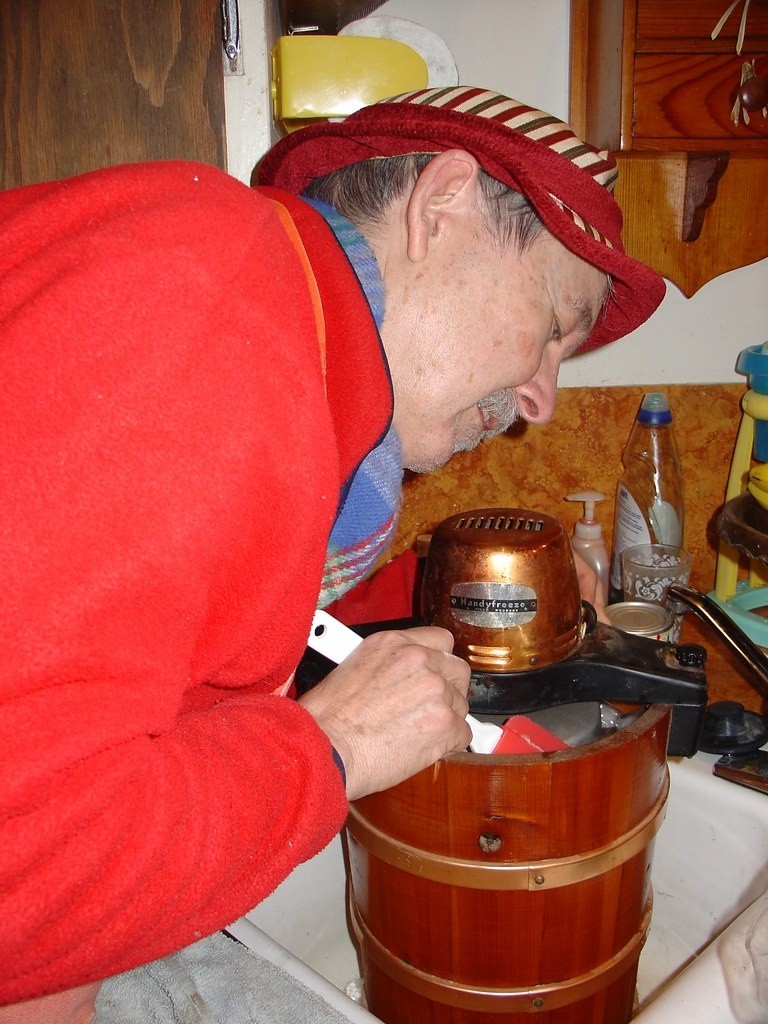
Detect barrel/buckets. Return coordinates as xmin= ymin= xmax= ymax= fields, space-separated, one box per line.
xmin=342 ymin=702 xmax=668 ymax=1024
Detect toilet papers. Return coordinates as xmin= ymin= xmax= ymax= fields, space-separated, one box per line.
xmin=327 ymin=16 xmax=459 ymax=123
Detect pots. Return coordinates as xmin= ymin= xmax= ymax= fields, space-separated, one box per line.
xmin=415 ymin=507 xmax=597 ymax=673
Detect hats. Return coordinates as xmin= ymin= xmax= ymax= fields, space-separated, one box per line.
xmin=250 ymin=86 xmax=667 ymax=361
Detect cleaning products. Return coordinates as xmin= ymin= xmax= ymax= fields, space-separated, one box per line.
xmin=610 ymin=391 xmax=685 ymax=604
xmin=564 ymin=491 xmax=609 ymax=624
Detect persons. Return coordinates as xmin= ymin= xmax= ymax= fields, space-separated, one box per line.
xmin=0 ymin=85 xmax=667 ymax=1024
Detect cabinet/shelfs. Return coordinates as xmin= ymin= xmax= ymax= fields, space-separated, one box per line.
xmin=571 ymin=0 xmax=768 ymax=300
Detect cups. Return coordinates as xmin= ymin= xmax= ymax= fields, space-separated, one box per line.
xmin=621 ymin=544 xmax=690 ymax=644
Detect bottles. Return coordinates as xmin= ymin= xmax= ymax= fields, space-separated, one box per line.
xmin=611 ymin=392 xmax=685 ymax=603
